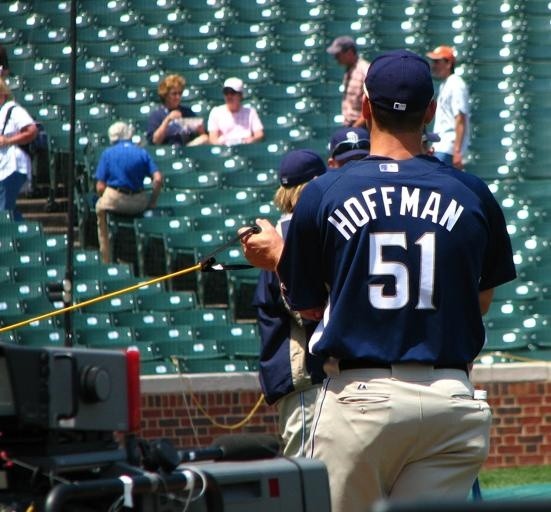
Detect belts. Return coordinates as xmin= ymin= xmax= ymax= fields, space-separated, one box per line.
xmin=338 ymin=358 xmax=467 ymax=371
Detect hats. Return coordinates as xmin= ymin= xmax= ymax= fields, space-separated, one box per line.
xmin=223 ymin=78 xmax=244 ymax=94
xmin=427 ymin=47 xmax=452 ymax=61
xmin=364 ymin=49 xmax=434 ymax=110
xmin=279 ymin=150 xmax=326 ymax=187
xmin=327 ymin=36 xmax=352 ymax=54
xmin=109 ymin=122 xmax=132 ymax=142
xmin=332 ymin=127 xmax=371 ymax=162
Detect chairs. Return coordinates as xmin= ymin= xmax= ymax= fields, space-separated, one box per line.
xmin=0 ymin=0 xmax=551 ymax=376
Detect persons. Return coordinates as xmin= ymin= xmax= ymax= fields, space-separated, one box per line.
xmin=256 ymin=149 xmax=329 ymax=459
xmin=424 ymin=43 xmax=470 ymax=170
xmin=147 ymin=74 xmax=208 ymax=147
xmin=0 ymin=80 xmax=39 ymax=221
xmin=236 ymin=52 xmax=493 ymax=510
xmin=422 ymin=127 xmax=515 ymax=318
xmin=207 ymin=76 xmax=264 ymax=146
xmin=326 ymin=127 xmax=370 ymax=171
xmin=96 ymin=120 xmax=163 ymax=263
xmin=326 ymin=36 xmax=370 ymax=132
